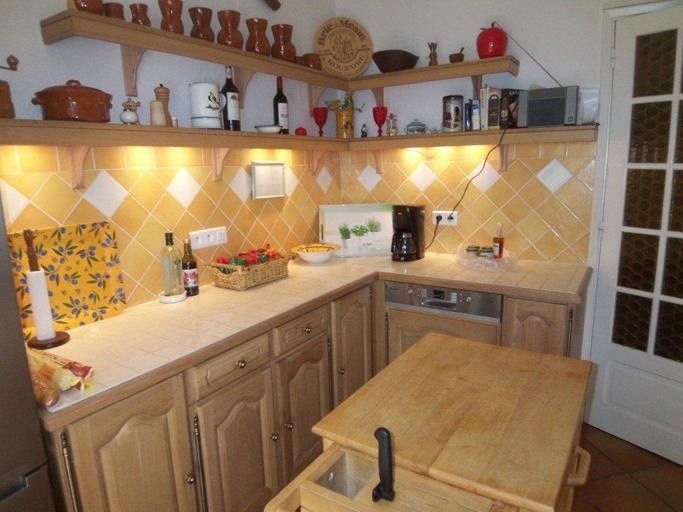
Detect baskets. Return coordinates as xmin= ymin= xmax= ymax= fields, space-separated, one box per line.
xmin=210 ymin=253 xmax=290 ymax=291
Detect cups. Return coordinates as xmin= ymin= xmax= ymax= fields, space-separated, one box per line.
xmin=187 ymin=82 xmax=221 ymax=129
xmin=442 ymin=95 xmax=480 ymax=132
xmin=337 ymin=110 xmax=354 ymax=138
xmin=447 ymin=52 xmax=464 ymax=65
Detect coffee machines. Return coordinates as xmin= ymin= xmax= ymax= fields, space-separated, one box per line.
xmin=391 ymin=204 xmax=425 ymax=261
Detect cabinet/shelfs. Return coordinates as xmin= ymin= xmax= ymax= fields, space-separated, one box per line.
xmin=43 ymin=372 xmax=199 ymax=510
xmin=501 ymin=297 xmax=586 ymax=360
xmin=1 ymin=11 xmax=598 ymax=152
xmin=329 ymin=286 xmax=373 ymax=410
xmin=184 ymin=303 xmax=333 ymax=511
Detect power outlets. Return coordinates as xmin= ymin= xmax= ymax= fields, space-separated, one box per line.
xmin=432 ymin=211 xmax=457 ymax=225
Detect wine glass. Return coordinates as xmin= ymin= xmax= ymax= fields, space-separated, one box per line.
xmin=372 ymin=107 xmax=387 ymax=137
xmin=312 ymin=107 xmax=327 ymax=136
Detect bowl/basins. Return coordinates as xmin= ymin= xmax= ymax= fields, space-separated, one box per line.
xmin=291 ymin=244 xmax=340 ymax=263
xmin=371 ymin=50 xmax=420 ymax=74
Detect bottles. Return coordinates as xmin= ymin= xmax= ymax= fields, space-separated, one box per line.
xmin=120 ymin=84 xmax=178 ymax=128
xmin=272 ymin=76 xmax=288 ymax=134
xmin=221 ymin=66 xmax=241 ymax=131
xmin=159 ymin=231 xmax=183 ymax=295
xmin=0 ymin=81 xmax=14 ymax=120
xmin=180 ymin=239 xmax=199 ymax=295
xmin=492 ymin=223 xmax=503 ymax=260
xmin=295 ymin=127 xmax=306 ymax=136
xmin=77 ymin=0 xmax=320 ymax=71
xmin=360 ymin=124 xmax=367 ymax=137
xmin=407 ymin=119 xmax=425 ymax=136
xmin=387 ymin=114 xmax=396 ymax=136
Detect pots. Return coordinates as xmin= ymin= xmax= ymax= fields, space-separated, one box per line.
xmin=31 ymin=80 xmax=114 ymax=122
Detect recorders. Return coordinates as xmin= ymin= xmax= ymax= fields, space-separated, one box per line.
xmin=517 ymin=86 xmax=579 ymax=128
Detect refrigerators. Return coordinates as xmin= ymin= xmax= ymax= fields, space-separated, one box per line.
xmin=0 ymin=197 xmax=55 ymax=512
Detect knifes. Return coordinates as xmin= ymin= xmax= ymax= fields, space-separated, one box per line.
xmin=371 ymin=427 xmax=393 ymax=500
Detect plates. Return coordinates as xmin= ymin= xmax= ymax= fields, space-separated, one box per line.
xmin=158 ymin=289 xmax=188 ymax=302
xmin=255 ymin=125 xmax=282 ymax=134
